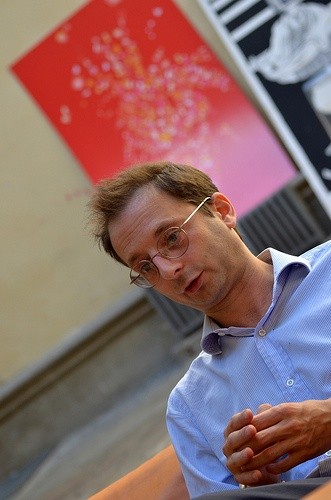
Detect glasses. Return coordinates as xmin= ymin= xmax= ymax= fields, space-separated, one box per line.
xmin=128 ymin=194 xmax=211 ymax=289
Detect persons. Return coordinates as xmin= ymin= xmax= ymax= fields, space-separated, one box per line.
xmin=90 ymin=163 xmax=331 ymax=500
